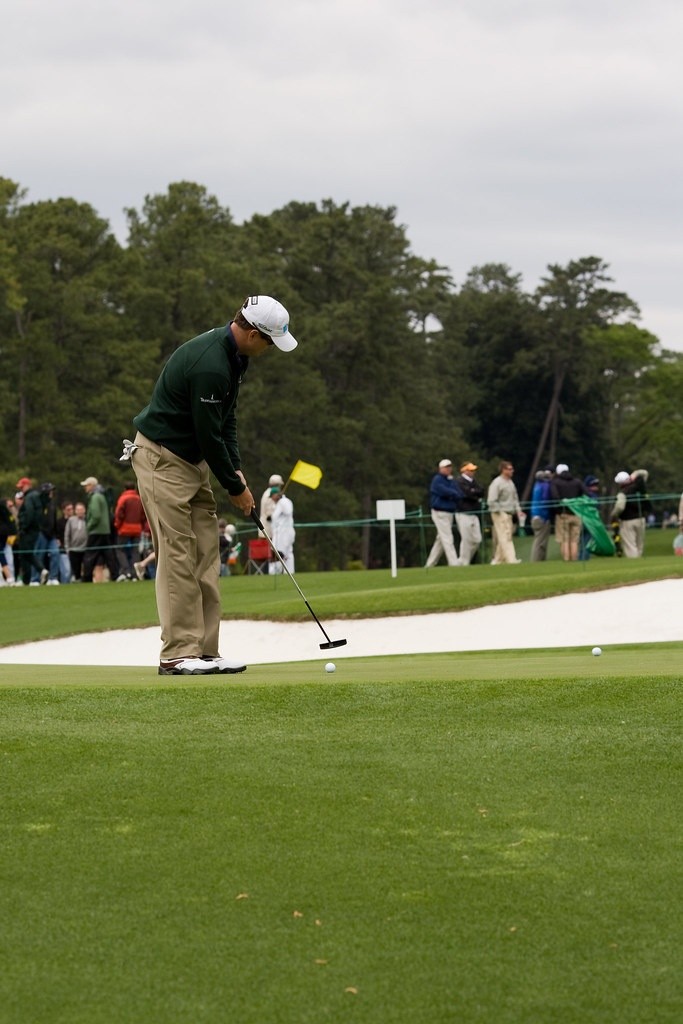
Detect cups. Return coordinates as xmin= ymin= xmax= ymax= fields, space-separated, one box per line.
xmin=519 ymin=515 xmax=526 ymax=527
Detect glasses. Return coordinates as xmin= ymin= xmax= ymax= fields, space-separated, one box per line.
xmin=503 ymin=467 xmax=513 ymax=470
xmin=255 ymin=328 xmax=275 ymax=346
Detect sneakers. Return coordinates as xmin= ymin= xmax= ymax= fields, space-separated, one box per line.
xmin=200 ymin=655 xmax=246 ymax=674
xmin=157 ymin=655 xmax=219 ymax=675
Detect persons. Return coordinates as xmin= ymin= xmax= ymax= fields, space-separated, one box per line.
xmin=0 ymin=474 xmax=296 ymax=587
xmin=454 ymin=461 xmax=486 ymax=567
xmin=487 ymin=460 xmax=522 ymax=565
xmin=424 ymin=459 xmax=467 ymax=566
xmin=529 ymin=463 xmax=653 ymax=561
xmin=131 ymin=295 xmax=300 ymax=673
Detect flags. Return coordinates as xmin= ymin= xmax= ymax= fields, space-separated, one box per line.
xmin=290 ymin=459 xmax=322 ymax=489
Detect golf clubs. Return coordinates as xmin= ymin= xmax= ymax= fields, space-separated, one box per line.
xmin=250 ymin=507 xmax=348 ymax=651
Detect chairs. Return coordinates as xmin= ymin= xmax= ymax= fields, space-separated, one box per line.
xmin=248 ymin=538 xmax=271 ymax=576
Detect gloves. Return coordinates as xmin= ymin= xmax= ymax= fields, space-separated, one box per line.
xmin=119 ymin=438 xmax=138 ymax=460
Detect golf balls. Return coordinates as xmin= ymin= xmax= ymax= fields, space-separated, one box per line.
xmin=325 ymin=662 xmax=336 ymax=672
xmin=592 ymin=646 xmax=602 ymax=656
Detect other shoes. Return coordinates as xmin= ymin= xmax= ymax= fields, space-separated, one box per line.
xmin=0 ymin=562 xmax=146 ymax=587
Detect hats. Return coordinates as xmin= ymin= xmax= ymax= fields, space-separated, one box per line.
xmin=439 ymin=459 xmax=451 ymax=468
xmin=556 ymin=464 xmax=568 ymax=475
xmin=41 ymin=482 xmax=55 ymax=492
xmin=241 ymin=295 xmax=298 ymax=352
xmin=16 ymin=491 xmax=24 ymax=499
xmin=79 ymin=477 xmax=98 ymax=486
xmin=461 ymin=463 xmax=478 ymax=473
xmin=268 ymin=487 xmax=282 ymax=498
xmin=614 ymin=471 xmax=629 ymax=483
xmin=16 ymin=478 xmax=31 ymax=488
xmin=269 ymin=474 xmax=285 ymax=486
xmin=584 ymin=474 xmax=599 ymax=487
